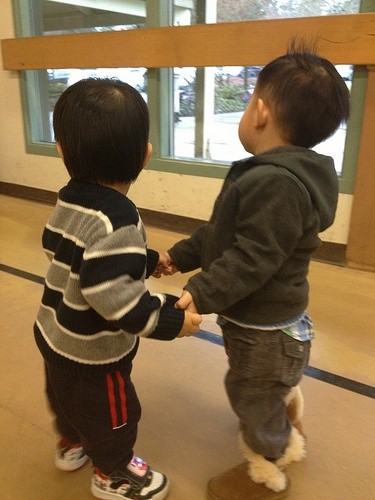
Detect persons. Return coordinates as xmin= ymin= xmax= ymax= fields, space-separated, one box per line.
xmin=157 ymin=53 xmax=351 ymax=500
xmin=32 ymin=77 xmax=201 ymax=500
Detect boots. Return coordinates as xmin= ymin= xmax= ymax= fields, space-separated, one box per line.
xmin=206 ymin=423 xmax=306 ymax=500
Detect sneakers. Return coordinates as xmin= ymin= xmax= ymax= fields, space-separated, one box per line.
xmin=90 ymin=456 xmax=170 ymax=500
xmin=55 ymin=438 xmax=89 ymax=470
